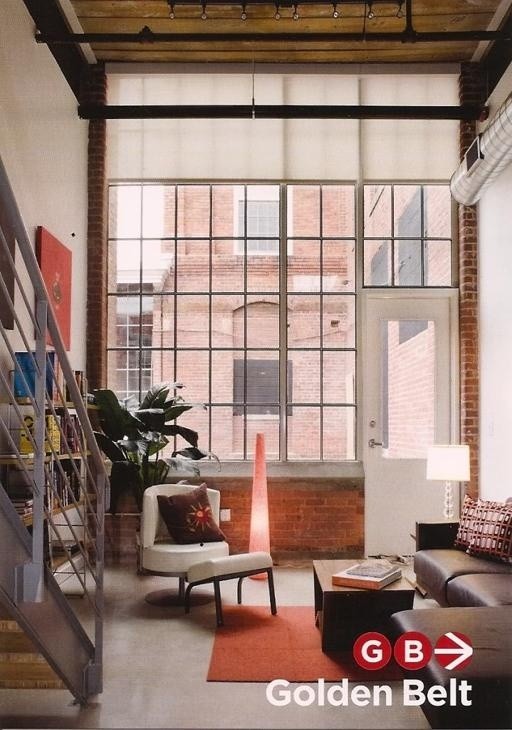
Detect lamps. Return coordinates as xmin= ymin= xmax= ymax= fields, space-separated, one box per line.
xmin=425 ymin=442 xmax=472 ymax=521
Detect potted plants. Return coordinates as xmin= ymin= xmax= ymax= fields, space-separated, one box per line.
xmin=91 ymin=380 xmax=223 ymax=578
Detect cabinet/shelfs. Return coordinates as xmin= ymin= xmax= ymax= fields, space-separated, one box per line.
xmin=0 ymin=369 xmax=90 ymax=588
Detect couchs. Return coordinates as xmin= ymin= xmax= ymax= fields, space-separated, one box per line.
xmin=182 ymin=549 xmax=277 ymax=628
xmin=139 ymin=482 xmax=232 ymax=607
xmin=394 ymin=520 xmax=512 ymax=730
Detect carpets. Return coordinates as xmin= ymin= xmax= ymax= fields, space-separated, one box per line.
xmin=203 ymin=603 xmax=410 ymax=684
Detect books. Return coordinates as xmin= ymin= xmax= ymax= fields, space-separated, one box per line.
xmin=331 ymin=558 xmax=402 ymax=589
xmin=14 ymin=349 xmax=88 ymax=400
xmin=51 ymin=539 xmax=83 ymax=556
xmin=5 ymin=457 xmax=83 ymax=514
xmin=20 ymin=408 xmax=85 ymax=453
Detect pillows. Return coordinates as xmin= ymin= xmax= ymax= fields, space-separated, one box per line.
xmin=155 ymin=483 xmax=227 ymax=542
xmin=467 ymin=496 xmax=512 ymax=566
xmin=455 ymin=496 xmax=476 ymax=550
